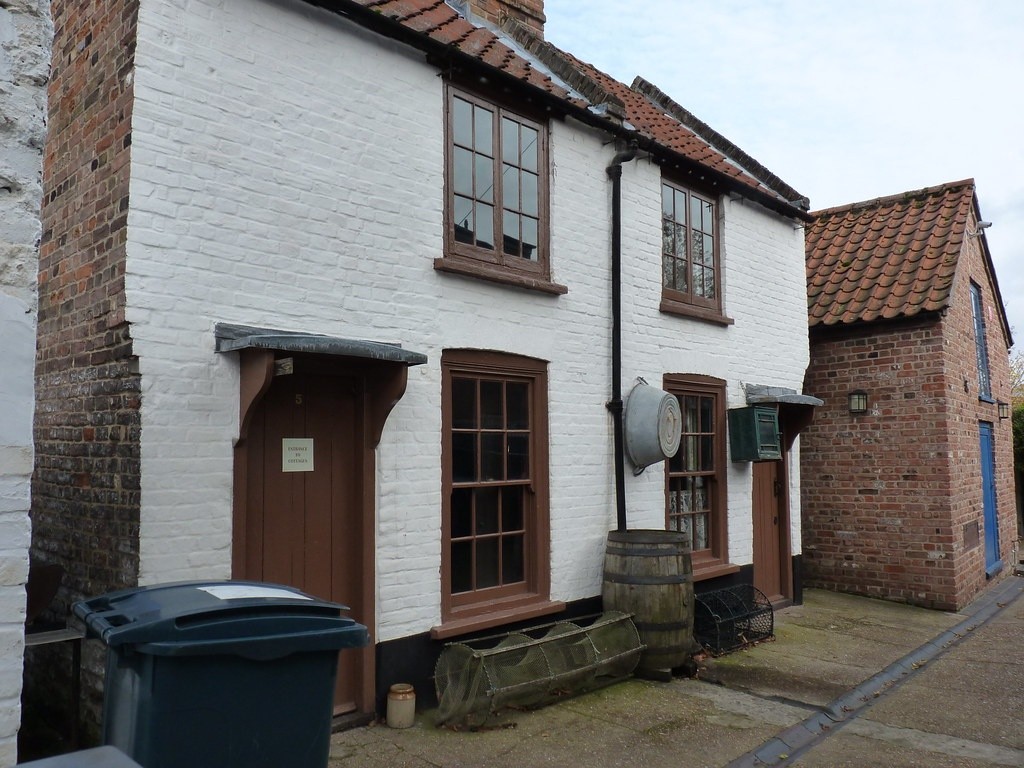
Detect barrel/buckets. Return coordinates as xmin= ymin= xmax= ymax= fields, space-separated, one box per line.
xmin=602 ymin=529 xmax=695 ymax=669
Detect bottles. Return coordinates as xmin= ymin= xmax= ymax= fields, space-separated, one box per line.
xmin=386 ymin=684 xmax=415 ymax=728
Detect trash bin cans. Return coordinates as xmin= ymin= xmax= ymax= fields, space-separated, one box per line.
xmin=71 ymin=580 xmax=370 ymax=768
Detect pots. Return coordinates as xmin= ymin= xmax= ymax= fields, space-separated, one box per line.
xmin=623 ymin=376 xmax=683 ymax=477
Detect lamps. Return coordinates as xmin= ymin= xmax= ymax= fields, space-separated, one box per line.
xmin=998 ymin=403 xmax=1009 ymax=419
xmin=848 ymin=389 xmax=868 ymax=413
